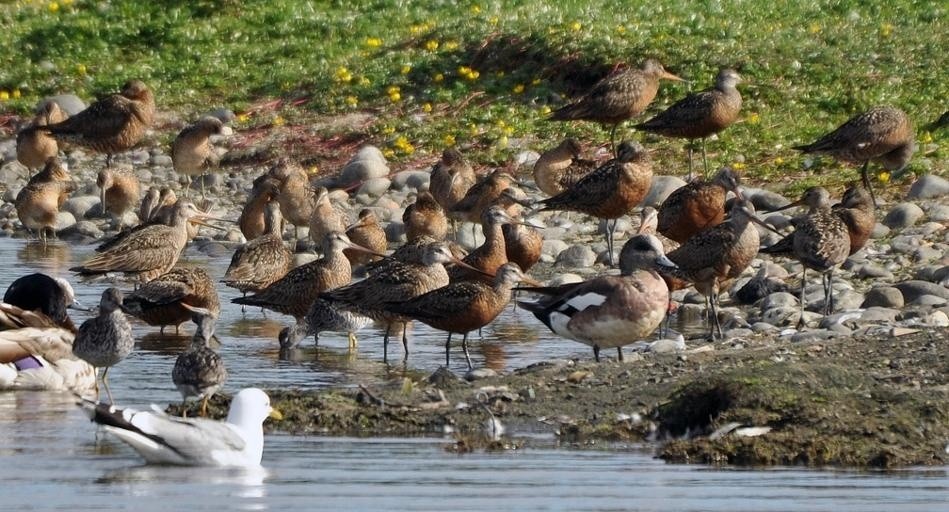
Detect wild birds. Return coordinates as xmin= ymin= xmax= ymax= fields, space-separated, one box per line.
xmin=72 ymin=386 xmax=284 ymax=469
xmin=791 ymin=105 xmax=915 ymax=209
xmin=0 ymin=57 xmax=876 ymax=420
